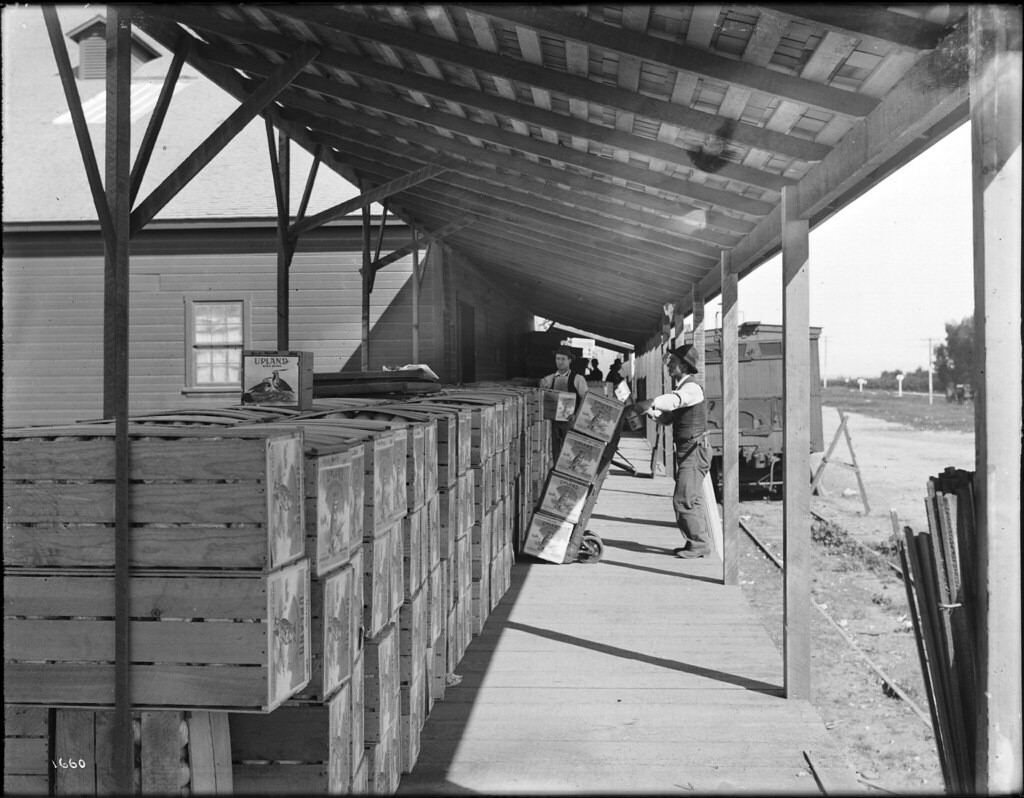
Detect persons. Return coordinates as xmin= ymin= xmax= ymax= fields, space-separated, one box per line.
xmin=538 ymin=345 xmax=589 ymax=464
xmin=583 ymin=358 xmax=625 ymax=386
xmin=631 ymin=343 xmax=714 ymax=558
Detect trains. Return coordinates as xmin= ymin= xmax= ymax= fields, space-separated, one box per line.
xmin=672 ymin=323 xmax=826 ymax=495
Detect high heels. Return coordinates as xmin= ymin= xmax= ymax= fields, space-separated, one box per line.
xmin=673 ymin=547 xmax=690 ymax=554
xmin=678 ymin=548 xmax=710 ymax=558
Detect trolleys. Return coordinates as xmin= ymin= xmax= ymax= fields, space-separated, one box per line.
xmin=563 ymin=402 xmax=651 ymax=564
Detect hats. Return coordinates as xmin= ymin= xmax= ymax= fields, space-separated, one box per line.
xmin=615 ymin=359 xmax=623 ymax=364
xmin=553 ymin=346 xmax=576 ymax=361
xmin=666 ymin=344 xmax=699 ymax=374
